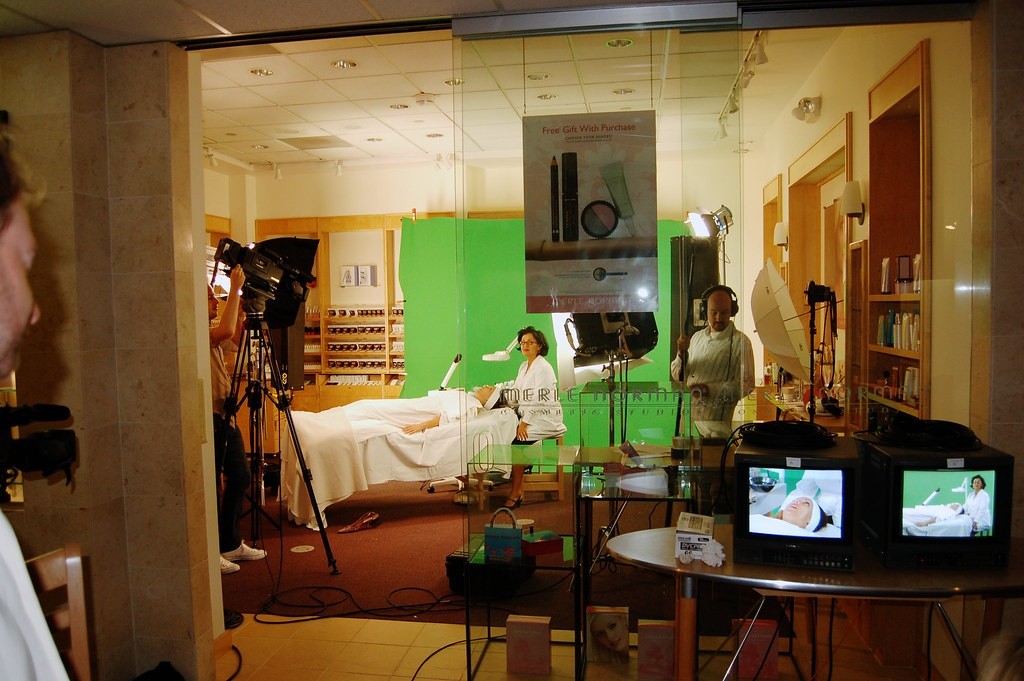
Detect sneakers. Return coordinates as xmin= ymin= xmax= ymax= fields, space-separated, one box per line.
xmin=221 ymin=538 xmax=267 ymax=562
xmin=220 ymin=554 xmax=240 ymax=575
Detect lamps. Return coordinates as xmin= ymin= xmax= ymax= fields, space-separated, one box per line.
xmin=686 ymin=203 xmax=735 ymax=285
xmin=788 ymin=96 xmax=822 ymax=124
xmin=838 ymin=180 xmax=866 ymax=222
xmin=752 ymin=35 xmax=768 ymax=67
xmin=724 ymin=93 xmax=743 ymax=115
xmin=271 ymin=163 xmax=284 ymax=182
xmin=732 ymin=63 xmax=754 ymax=90
xmin=333 ymin=159 xmax=346 ymax=178
xmin=434 ymin=354 xmax=463 ymax=390
xmin=719 ymin=118 xmax=729 ymax=141
xmin=482 ymin=335 xmax=518 ymax=361
xmin=772 ymin=221 xmax=788 ymax=252
xmin=203 ymin=147 xmax=219 ymax=170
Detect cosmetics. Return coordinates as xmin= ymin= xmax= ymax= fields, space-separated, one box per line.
xmin=874 ymin=364 xmax=919 ymax=406
xmin=874 ymin=306 xmax=921 ymax=354
xmin=877 ymin=255 xmax=923 ymax=293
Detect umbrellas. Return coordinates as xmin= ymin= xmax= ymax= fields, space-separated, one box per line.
xmin=751 ymin=253 xmax=846 ymax=387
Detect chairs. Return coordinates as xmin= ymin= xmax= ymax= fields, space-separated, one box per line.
xmin=21 ymin=540 xmax=96 ymax=681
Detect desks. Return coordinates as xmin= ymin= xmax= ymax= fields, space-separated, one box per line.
xmin=605 ymin=521 xmax=1024 ymax=681
xmin=763 ymin=391 xmax=846 ymax=432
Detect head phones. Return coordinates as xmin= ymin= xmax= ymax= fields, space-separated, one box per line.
xmin=699 ymin=284 xmax=739 ymax=320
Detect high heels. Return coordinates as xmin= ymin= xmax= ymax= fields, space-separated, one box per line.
xmin=524 ymin=465 xmax=533 ymax=474
xmin=499 ymin=494 xmax=523 ymax=512
xmin=337 ymin=512 xmax=380 ymax=533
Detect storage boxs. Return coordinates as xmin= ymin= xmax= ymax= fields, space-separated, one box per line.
xmin=731 ymin=617 xmax=780 ymax=680
xmin=506 ymin=615 xmax=552 ymax=676
xmin=358 ymin=265 xmax=378 ymax=288
xmin=635 ymin=619 xmax=675 ymax=681
xmin=585 ymin=605 xmax=630 ymax=663
xmin=674 ymin=510 xmax=715 ymax=560
xmin=340 ymin=264 xmax=357 ymax=288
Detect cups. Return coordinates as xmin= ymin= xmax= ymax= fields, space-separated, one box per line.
xmin=516 ymin=519 xmax=535 ymax=534
xmin=782 ymin=387 xmax=794 ymax=401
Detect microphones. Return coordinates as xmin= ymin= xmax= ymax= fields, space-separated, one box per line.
xmin=10 ymin=404 xmax=71 ymax=427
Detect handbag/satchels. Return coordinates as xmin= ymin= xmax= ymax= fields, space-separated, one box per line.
xmin=484 ymin=508 xmax=522 ymax=565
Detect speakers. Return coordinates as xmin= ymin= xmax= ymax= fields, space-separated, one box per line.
xmin=573 ymin=312 xmax=658 ymax=350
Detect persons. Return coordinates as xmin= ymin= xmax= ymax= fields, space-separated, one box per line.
xmin=749 ymin=494 xmax=828 ymax=538
xmin=206 ymin=264 xmax=268 ymax=572
xmin=403 ymin=384 xmax=510 ymax=435
xmin=499 ymin=325 xmax=567 ymax=510
xmin=773 ymin=469 xmax=841 ymax=527
xmin=961 ymin=474 xmax=990 ymax=536
xmin=667 ymin=284 xmax=758 ymax=441
xmin=1 ymin=107 xmax=72 ymax=679
xmin=903 ymin=500 xmax=966 ymax=527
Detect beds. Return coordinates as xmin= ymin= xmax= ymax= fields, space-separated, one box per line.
xmin=282 ymin=407 xmax=518 ymax=521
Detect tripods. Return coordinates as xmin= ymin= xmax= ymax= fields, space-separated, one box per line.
xmin=225 ymin=280 xmax=345 ymax=578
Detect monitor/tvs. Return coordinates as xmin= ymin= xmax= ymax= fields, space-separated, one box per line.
xmin=733 ymin=434 xmax=857 ymax=573
xmin=856 ymin=434 xmax=1014 ymax=571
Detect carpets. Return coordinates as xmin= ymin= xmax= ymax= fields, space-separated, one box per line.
xmin=213 ymin=470 xmax=795 ymax=640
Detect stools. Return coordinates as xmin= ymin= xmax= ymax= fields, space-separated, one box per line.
xmin=484 ymin=430 xmax=565 ymax=502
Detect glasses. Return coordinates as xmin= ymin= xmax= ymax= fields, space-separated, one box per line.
xmin=520 ymin=340 xmax=538 ymax=345
xmin=973 ymin=480 xmax=982 ymax=484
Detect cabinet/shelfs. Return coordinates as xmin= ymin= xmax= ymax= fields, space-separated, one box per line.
xmin=204 ymin=211 xmax=525 ymax=385
xmin=464 ymin=444 xmax=911 ymax=681
xmin=846 ymin=239 xmax=932 ymax=430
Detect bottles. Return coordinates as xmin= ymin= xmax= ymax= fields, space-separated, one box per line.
xmin=580 ymin=466 xmax=605 ymax=496
xmin=763 ymin=362 xmax=792 ymax=385
xmin=678 ymin=466 xmax=698 ymax=499
